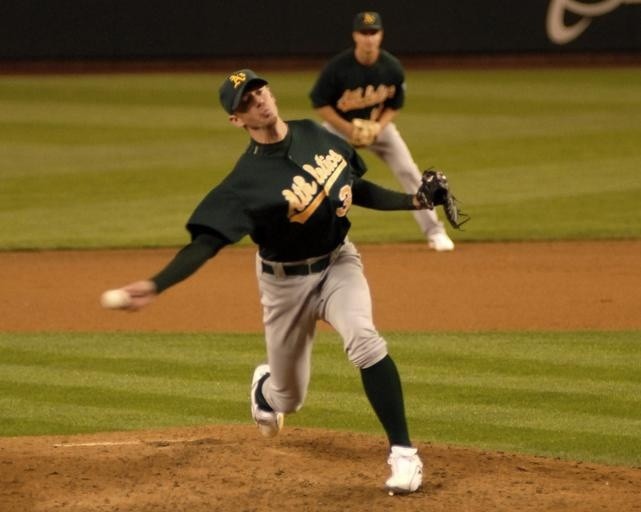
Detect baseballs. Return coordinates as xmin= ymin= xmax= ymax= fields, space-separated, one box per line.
xmin=99 ymin=288 xmax=130 ymax=310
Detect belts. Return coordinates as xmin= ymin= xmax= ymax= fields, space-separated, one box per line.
xmin=261 ymin=242 xmax=344 ymax=275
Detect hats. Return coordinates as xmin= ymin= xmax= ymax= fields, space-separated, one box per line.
xmin=353 ymin=11 xmax=382 ymax=31
xmin=219 ymin=69 xmax=268 ymax=114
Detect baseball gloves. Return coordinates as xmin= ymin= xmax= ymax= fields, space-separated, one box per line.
xmin=418 ymin=167 xmax=471 ymax=227
xmin=351 ymin=119 xmax=379 ymax=145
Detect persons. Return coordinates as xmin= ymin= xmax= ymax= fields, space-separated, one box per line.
xmin=122 ymin=70 xmax=449 ymax=493
xmin=310 ymin=12 xmax=456 ymax=251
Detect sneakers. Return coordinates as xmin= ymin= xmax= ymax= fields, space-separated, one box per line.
xmin=385 ymin=445 xmax=424 ymax=492
xmin=251 ymin=365 xmax=284 ymax=438
xmin=428 ymin=232 xmax=453 ymax=251
